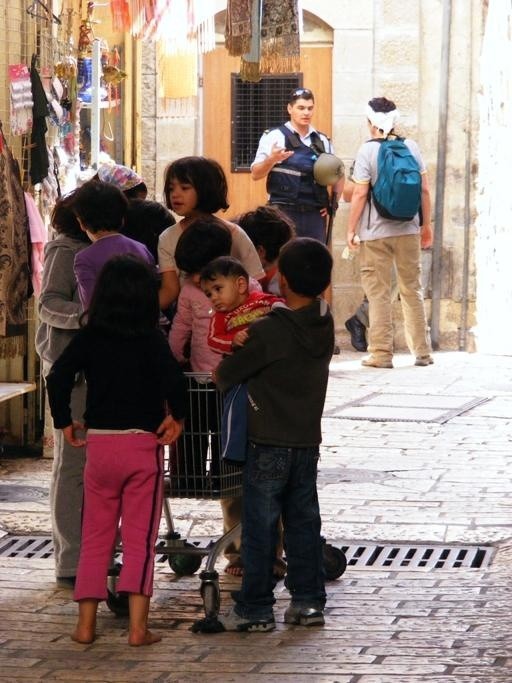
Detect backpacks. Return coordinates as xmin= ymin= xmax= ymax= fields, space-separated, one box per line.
xmin=366 ymin=135 xmax=423 ymax=230
xmin=265 ymin=124 xmax=330 ymax=209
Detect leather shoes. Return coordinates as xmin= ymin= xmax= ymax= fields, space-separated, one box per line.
xmin=56 ymin=574 xmax=74 ymax=589
xmin=345 ymin=316 xmax=368 ymax=352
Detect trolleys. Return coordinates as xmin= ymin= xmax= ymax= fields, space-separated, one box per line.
xmin=102 ymin=369 xmax=348 ymax=635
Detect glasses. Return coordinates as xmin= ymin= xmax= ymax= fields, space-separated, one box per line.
xmin=293 ymin=88 xmax=312 ymax=96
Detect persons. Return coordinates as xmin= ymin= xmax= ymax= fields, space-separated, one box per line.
xmin=222 ymin=205 xmax=296 ymax=578
xmin=90 ymin=163 xmax=148 ymax=202
xmin=341 ymin=160 xmax=399 ymax=352
xmin=72 ymin=180 xmax=155 ymax=323
xmin=346 ymin=97 xmax=435 ymax=369
xmin=216 ymin=236 xmax=335 ymax=634
xmin=198 ymin=255 xmax=284 ymax=352
xmin=33 ymin=183 xmax=124 ymax=588
xmin=157 ymin=156 xmax=269 ymax=309
xmin=45 ymin=252 xmax=190 ymax=647
xmin=250 ymin=88 xmax=346 ymax=355
xmin=168 ymin=218 xmax=263 ymax=496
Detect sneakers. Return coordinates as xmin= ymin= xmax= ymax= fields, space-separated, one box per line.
xmin=283 ymin=600 xmax=325 ymax=626
xmin=415 ymin=354 xmax=434 ymax=366
xmin=216 ymin=603 xmax=277 ymax=632
xmin=361 ymin=354 xmax=394 ymax=369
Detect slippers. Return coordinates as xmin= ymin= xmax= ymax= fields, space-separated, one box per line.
xmin=226 ymin=559 xmax=242 ymax=575
